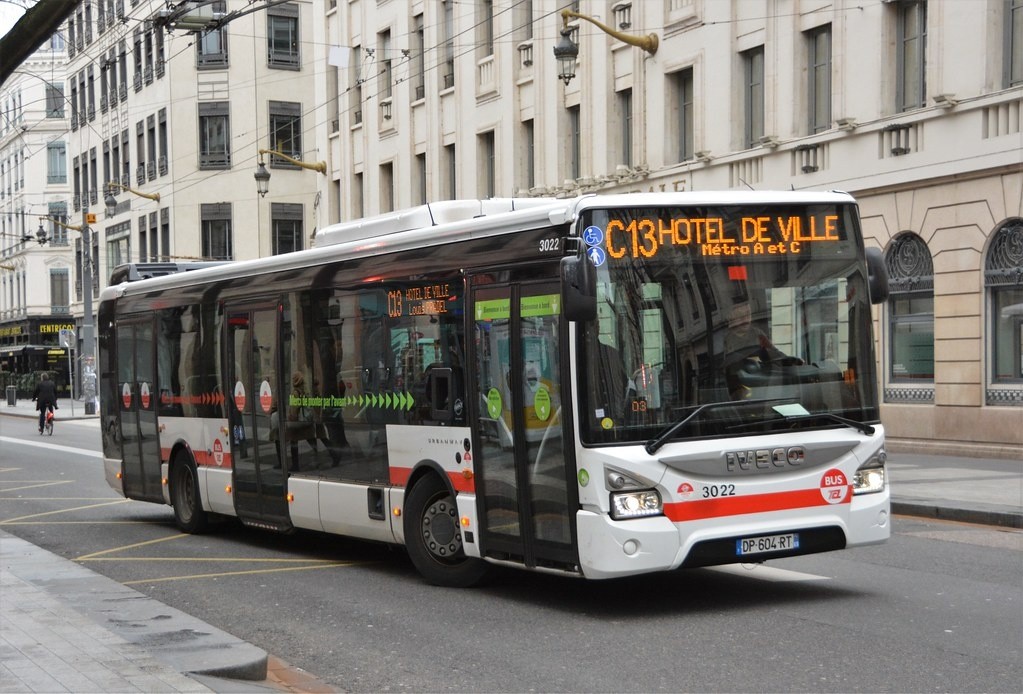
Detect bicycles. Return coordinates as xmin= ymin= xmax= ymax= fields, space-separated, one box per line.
xmin=31 ymin=398 xmax=55 ymax=436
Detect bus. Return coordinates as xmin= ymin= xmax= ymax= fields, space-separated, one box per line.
xmin=95 ymin=189 xmax=892 ymax=590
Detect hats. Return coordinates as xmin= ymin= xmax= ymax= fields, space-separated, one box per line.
xmin=291 ymin=371 xmax=304 ymax=386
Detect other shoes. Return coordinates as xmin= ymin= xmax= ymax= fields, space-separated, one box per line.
xmin=287 ymin=464 xmax=300 ymax=472
xmin=38 ymin=429 xmax=43 ymax=433
xmin=330 ymin=454 xmax=341 ymax=467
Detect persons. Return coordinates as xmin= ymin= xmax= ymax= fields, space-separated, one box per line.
xmin=32 ymin=373 xmax=57 ymax=433
xmin=270 ymin=372 xmax=341 ymax=474
xmin=724 ymin=304 xmax=805 ymax=367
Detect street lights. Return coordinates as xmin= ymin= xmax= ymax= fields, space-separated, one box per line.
xmin=36 ymin=217 xmax=98 ymax=415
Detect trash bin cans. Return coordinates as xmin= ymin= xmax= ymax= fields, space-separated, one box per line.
xmin=7 ymin=385 xmax=16 ymax=407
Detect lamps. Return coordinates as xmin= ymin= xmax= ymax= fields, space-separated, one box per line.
xmin=253 ymin=149 xmax=327 ymax=198
xmin=553 ymin=8 xmax=659 ymax=88
xmin=35 ymin=217 xmax=83 ymax=247
xmin=104 ymin=182 xmax=160 ymax=217
